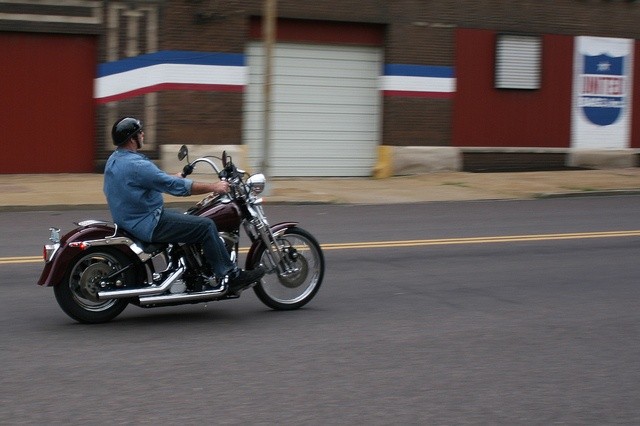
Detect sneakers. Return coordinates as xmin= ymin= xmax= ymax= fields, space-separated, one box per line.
xmin=229 ymin=267 xmax=265 ymax=293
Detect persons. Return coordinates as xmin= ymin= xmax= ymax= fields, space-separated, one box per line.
xmin=103 ymin=117 xmax=266 ymax=292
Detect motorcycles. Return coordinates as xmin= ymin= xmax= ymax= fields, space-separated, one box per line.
xmin=37 ymin=145 xmax=325 ymax=324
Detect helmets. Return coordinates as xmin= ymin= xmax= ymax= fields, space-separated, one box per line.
xmin=112 ymin=117 xmax=142 ymax=146
